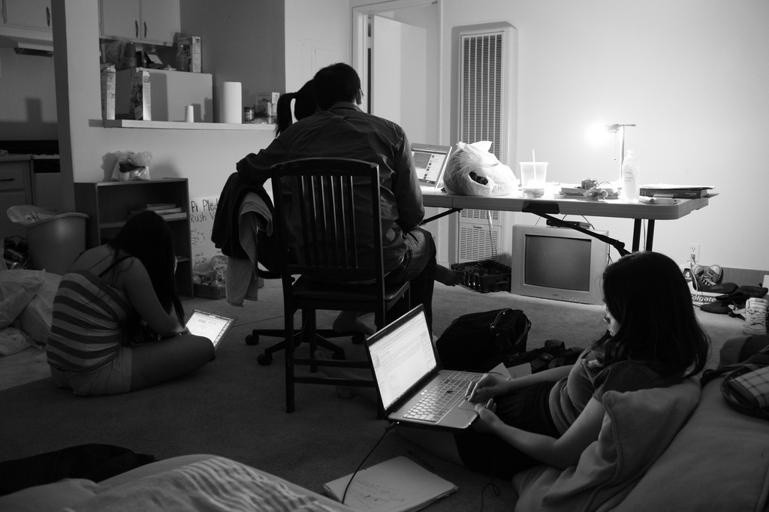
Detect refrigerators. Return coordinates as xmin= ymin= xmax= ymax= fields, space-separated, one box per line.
xmin=116 ymin=66 xmax=215 ymax=123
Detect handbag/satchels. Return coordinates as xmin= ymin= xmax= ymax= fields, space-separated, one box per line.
xmin=436 ymin=308 xmax=531 ymax=372
xmin=726 ymin=364 xmax=769 ymax=410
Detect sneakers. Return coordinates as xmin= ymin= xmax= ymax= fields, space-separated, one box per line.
xmin=691 ymin=265 xmax=724 ymax=292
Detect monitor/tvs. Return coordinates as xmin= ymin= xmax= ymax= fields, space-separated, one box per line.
xmin=510 ymin=224 xmax=609 ymax=305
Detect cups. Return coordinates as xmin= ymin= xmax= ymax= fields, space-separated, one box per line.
xmin=522 ymin=162 xmax=547 ymax=198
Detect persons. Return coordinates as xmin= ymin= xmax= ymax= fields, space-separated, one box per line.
xmin=258 ymin=62 xmax=435 ymax=350
xmin=276 ymin=78 xmax=460 ymax=334
xmin=466 ymin=251 xmax=712 ymax=490
xmin=46 ymin=210 xmax=216 ymax=397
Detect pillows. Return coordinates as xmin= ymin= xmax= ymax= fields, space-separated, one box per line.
xmin=512 ymin=376 xmax=702 ymax=512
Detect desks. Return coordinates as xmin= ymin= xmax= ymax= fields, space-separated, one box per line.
xmin=416 ymin=187 xmax=707 ymax=257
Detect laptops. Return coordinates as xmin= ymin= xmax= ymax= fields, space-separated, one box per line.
xmin=363 ymin=303 xmax=511 ymax=430
xmin=410 ymin=143 xmax=453 ymax=191
xmin=185 ymin=309 xmax=235 ymax=351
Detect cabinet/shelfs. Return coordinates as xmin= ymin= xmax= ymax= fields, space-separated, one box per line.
xmin=99 ymin=0 xmax=181 ymax=49
xmin=1 ymin=154 xmax=36 ymax=243
xmin=74 ymin=178 xmax=194 ymax=301
xmin=0 ymin=0 xmax=54 ymax=55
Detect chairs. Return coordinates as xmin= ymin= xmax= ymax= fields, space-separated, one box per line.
xmin=230 ymin=172 xmax=365 ymax=364
xmin=270 ymin=159 xmax=410 ymax=413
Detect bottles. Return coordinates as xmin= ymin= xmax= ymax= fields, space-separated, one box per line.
xmin=184 ymin=105 xmax=194 ymax=123
xmin=622 ymin=154 xmax=640 ymax=204
xmin=245 ymin=104 xmax=255 ymax=124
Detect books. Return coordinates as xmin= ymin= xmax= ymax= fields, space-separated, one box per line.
xmin=640 ymin=187 xmax=718 ymax=200
